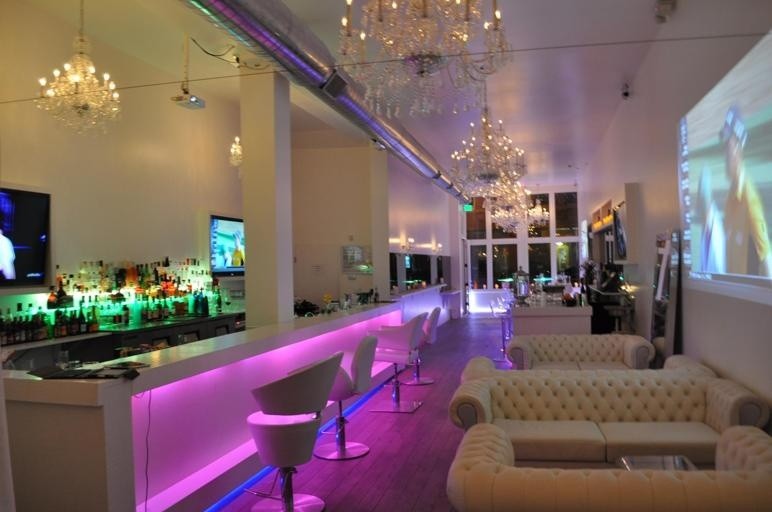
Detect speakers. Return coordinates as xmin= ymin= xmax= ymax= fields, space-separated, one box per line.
xmin=322 ymin=70 xmax=349 ymax=102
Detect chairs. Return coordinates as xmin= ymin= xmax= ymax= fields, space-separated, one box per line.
xmin=367 ymin=311 xmax=429 ymax=414
xmin=484 ymin=294 xmax=516 ymax=363
xmin=381 ymin=305 xmax=442 ymax=387
xmin=244 ymin=351 xmax=344 ymax=512
xmin=312 ymin=334 xmax=378 ymax=461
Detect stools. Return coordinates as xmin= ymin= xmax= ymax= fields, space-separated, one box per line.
xmin=607 ymin=306 xmax=633 ymax=332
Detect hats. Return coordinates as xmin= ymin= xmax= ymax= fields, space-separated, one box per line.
xmin=233 ymin=230 xmax=242 ymax=242
xmin=719 ymin=107 xmax=748 ymax=149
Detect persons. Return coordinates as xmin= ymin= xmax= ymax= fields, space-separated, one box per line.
xmin=232 ymin=231 xmax=245 ymax=266
xmin=716 ymin=102 xmax=770 ymax=277
xmin=697 ymin=168 xmax=723 ymax=276
xmin=1 ymin=193 xmax=18 ymax=281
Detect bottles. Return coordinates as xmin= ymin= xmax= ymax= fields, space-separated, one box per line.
xmin=0 ymin=253 xmax=243 ymax=347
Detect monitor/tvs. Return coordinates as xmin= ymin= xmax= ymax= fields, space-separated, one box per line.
xmin=206 ymin=212 xmax=245 ymax=278
xmin=0 ymin=184 xmax=53 ymax=296
xmin=405 ymin=254 xmax=431 ymax=285
xmin=389 ymin=251 xmax=398 ymax=286
xmin=437 ymin=255 xmax=454 ymax=290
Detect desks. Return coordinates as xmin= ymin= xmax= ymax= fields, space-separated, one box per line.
xmin=620 ymin=454 xmax=700 ymax=472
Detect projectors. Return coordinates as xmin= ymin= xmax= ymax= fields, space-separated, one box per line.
xmin=167 ymin=91 xmax=206 ymax=110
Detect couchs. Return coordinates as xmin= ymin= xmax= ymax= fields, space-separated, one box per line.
xmin=448 ymin=422 xmax=772 ymax=510
xmin=501 ymin=332 xmax=656 ymax=372
xmin=450 ymin=379 xmax=771 ymax=471
xmin=460 ymin=353 xmax=719 ymax=384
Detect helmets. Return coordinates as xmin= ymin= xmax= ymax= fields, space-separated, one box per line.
xmin=1 ymin=194 xmax=15 ymax=232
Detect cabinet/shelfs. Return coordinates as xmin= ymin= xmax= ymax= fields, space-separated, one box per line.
xmin=66 ymin=312 xmax=238 ymax=362
xmin=587 ymin=182 xmax=640 ymax=268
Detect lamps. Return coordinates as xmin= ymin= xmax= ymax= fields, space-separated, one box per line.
xmin=228 ymin=135 xmax=242 ymax=179
xmin=29 ymin=1 xmax=126 ymax=140
xmin=330 ymin=0 xmax=511 ymax=119
xmin=452 ymin=80 xmax=550 ymax=242
